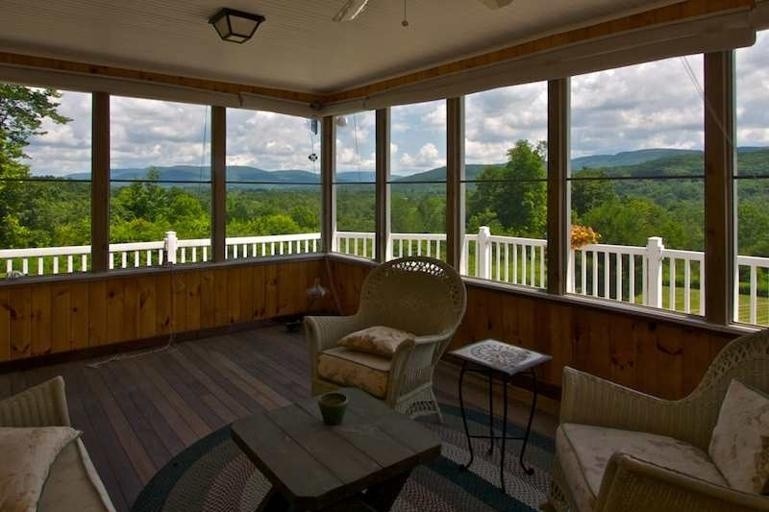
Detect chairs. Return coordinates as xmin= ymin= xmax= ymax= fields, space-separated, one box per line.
xmin=546 ymin=328 xmax=769 ymax=511
xmin=302 ymin=256 xmax=469 ymax=424
xmin=0 ymin=375 xmax=120 ymax=512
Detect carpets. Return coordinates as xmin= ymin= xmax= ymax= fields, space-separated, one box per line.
xmin=129 ymin=402 xmax=580 ymax=512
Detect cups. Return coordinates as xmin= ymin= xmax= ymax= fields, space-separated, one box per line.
xmin=318 ymin=392 xmax=349 ymax=424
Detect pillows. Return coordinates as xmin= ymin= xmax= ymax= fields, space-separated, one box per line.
xmin=334 ymin=325 xmax=416 ymax=361
xmin=0 ymin=425 xmax=84 ymax=512
xmin=707 ymin=378 xmax=769 ymax=496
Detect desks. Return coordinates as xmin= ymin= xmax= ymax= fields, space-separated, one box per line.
xmin=229 ymin=387 xmax=442 ymax=512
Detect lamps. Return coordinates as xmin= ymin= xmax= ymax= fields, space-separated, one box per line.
xmin=208 ymin=8 xmax=266 ymax=45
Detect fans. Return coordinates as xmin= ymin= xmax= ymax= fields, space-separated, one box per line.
xmin=332 ymin=0 xmax=515 ymax=27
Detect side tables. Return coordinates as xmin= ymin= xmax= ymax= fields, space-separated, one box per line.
xmin=447 ymin=339 xmax=554 ymax=494
